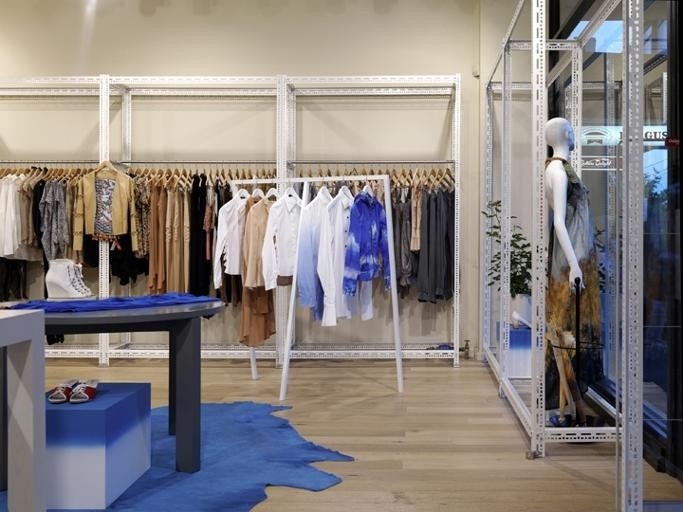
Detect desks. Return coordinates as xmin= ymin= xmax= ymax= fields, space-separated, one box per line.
xmin=0 ymin=296 xmax=225 ymax=473
xmin=0 ymin=308 xmax=46 ymax=511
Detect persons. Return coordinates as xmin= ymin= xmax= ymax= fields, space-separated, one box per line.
xmin=543 ymin=117 xmax=602 ymax=426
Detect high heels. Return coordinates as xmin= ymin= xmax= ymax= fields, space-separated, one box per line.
xmin=45 ymin=259 xmax=92 ymax=299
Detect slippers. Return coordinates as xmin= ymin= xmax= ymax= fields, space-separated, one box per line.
xmin=48 ymin=379 xmax=79 ymax=403
xmin=70 ymin=379 xmax=98 ymax=403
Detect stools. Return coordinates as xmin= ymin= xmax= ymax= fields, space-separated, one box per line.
xmin=46 ymin=383 xmax=151 ymax=509
xmin=496 ymin=321 xmax=532 ymax=379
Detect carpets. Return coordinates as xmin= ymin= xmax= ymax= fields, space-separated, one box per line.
xmin=0 ymin=401 xmax=356 ymax=512
